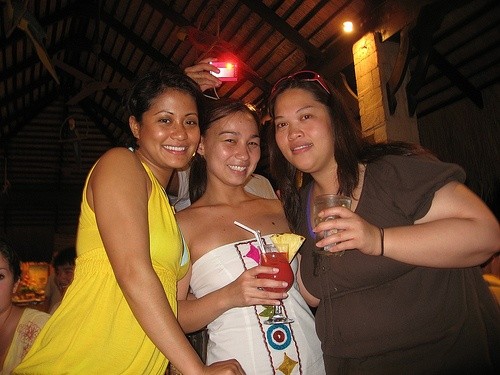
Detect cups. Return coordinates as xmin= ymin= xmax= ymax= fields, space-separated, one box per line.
xmin=314 ymin=194 xmax=351 ymax=257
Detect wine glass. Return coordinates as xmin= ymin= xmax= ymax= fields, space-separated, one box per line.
xmin=257 ymin=244 xmax=294 ymax=324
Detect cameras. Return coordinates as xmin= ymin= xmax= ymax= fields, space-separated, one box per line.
xmin=209 ymin=60 xmax=238 ymax=81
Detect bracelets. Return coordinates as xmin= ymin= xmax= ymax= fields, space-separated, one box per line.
xmin=379 ymin=228 xmax=385 ymax=259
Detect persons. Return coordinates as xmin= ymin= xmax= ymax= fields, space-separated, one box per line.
xmin=8 ymin=66 xmax=248 ymax=375
xmin=171 ymin=99 xmax=323 ymax=375
xmin=1 ymin=245 xmax=54 ymax=375
xmin=267 ymin=71 xmax=500 ymax=375
xmin=50 ymin=246 xmax=77 ymax=317
xmin=159 ymin=57 xmax=282 ymax=375
